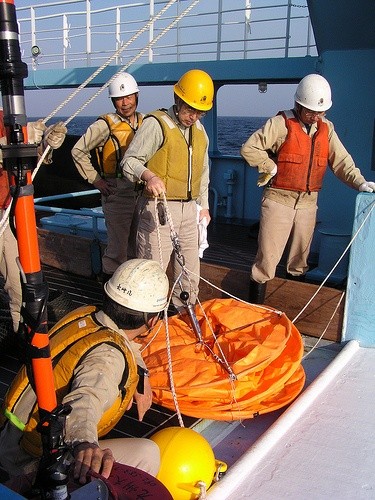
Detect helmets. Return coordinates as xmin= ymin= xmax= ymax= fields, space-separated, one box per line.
xmin=174 ymin=69 xmax=214 ymax=111
xmin=108 ymin=72 xmax=140 ymax=98
xmin=294 ymin=73 xmax=333 ymax=112
xmin=104 ymin=258 xmax=170 ymax=313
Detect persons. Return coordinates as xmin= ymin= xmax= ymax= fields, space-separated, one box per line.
xmin=71 ymin=72 xmax=146 ymax=284
xmin=0 ymin=260 xmax=169 ymax=500
xmin=120 ymin=70 xmax=214 ymax=320
xmin=0 ymin=111 xmax=67 ymax=334
xmin=240 ymin=74 xmax=375 ymax=305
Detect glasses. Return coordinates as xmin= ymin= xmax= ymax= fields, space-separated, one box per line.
xmin=181 ymin=103 xmax=206 ymax=118
xmin=301 ymin=108 xmax=326 ymax=118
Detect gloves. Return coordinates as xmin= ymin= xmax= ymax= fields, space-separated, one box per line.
xmin=27 ymin=119 xmax=47 ymax=144
xmin=359 ymin=181 xmax=375 ymax=193
xmin=256 ymin=164 xmax=277 ymax=187
xmin=46 ymin=121 xmax=67 ymax=149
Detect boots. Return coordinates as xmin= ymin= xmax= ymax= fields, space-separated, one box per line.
xmin=286 ymin=272 xmax=306 ymax=283
xmin=249 ymin=278 xmax=267 ymax=305
